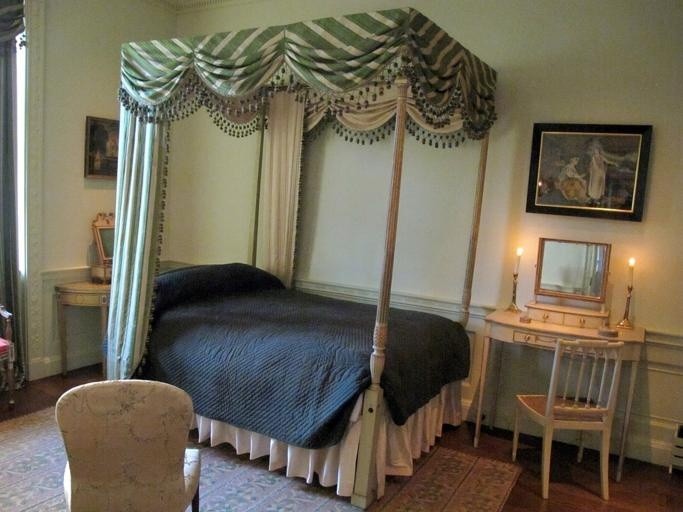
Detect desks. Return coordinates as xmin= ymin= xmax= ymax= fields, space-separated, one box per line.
xmin=473 ymin=307 xmax=646 ymax=483
xmin=56 ymin=281 xmax=112 ymax=379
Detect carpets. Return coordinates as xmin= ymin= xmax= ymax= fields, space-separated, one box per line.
xmin=0 ymin=404 xmax=522 ymax=512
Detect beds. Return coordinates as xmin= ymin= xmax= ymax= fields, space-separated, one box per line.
xmin=108 ymin=8 xmax=499 ymax=510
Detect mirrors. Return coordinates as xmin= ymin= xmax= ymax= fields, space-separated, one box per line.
xmin=92 ymin=211 xmax=114 ymax=266
xmin=535 ymin=238 xmax=611 ymax=304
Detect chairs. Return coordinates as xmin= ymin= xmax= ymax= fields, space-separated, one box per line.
xmin=0 ymin=304 xmax=19 ymax=411
xmin=56 ymin=379 xmax=201 ymax=512
xmin=513 ymin=338 xmax=624 ymax=500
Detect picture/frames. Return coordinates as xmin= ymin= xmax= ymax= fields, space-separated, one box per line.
xmin=84 ymin=116 xmax=120 ymax=180
xmin=526 ymin=121 xmax=653 ymax=222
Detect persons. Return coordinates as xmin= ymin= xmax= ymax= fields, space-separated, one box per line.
xmin=554 ymin=152 xmax=589 ymax=201
xmin=586 ymin=145 xmax=608 ymax=205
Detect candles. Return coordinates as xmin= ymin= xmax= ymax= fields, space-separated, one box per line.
xmin=512 ymin=249 xmax=522 ymax=275
xmin=626 ymin=257 xmax=635 ymax=287
